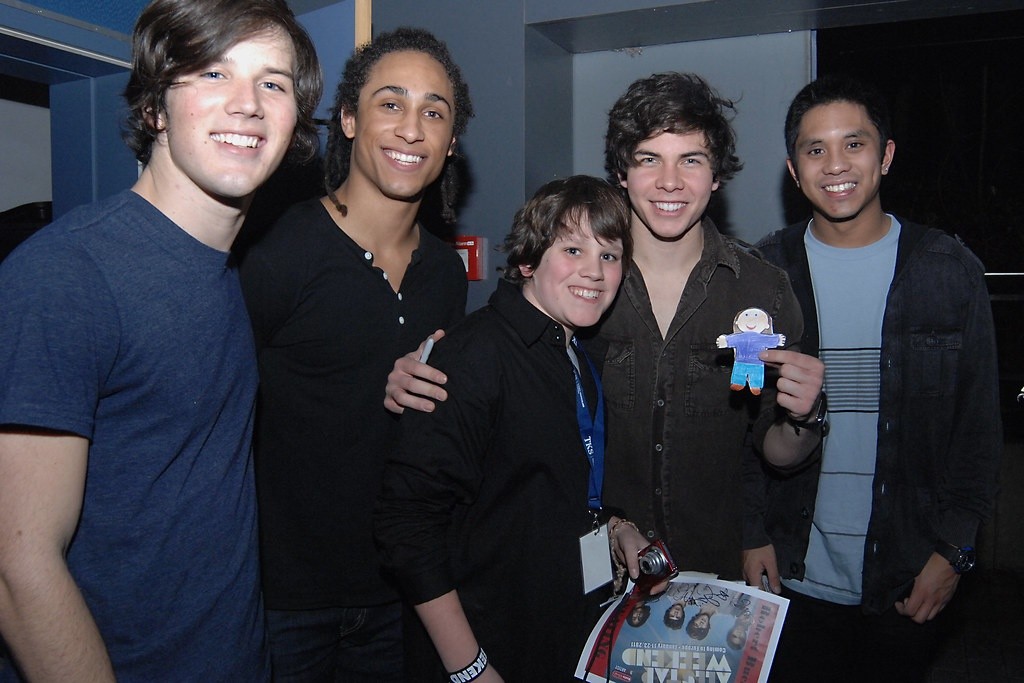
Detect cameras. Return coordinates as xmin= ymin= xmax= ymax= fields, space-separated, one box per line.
xmin=635 ymin=538 xmax=679 ymax=591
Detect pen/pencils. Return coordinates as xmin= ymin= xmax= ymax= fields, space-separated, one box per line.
xmin=419 ymin=338 xmax=436 ymax=365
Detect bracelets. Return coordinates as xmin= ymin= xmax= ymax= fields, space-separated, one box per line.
xmin=610 ymin=519 xmax=639 ymax=571
xmin=445 ymin=646 xmax=488 ymax=683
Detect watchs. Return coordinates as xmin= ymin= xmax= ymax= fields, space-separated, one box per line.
xmin=784 ymin=391 xmax=828 ymax=432
xmin=934 ymin=538 xmax=976 ymax=574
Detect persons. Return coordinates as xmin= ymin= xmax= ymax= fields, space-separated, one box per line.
xmin=0 ymin=0 xmax=325 ymax=683
xmin=242 ymin=26 xmax=470 ymax=683
xmin=625 ymin=582 xmax=756 ymax=649
xmin=756 ymin=74 xmax=999 ymax=683
xmin=383 ymin=71 xmax=829 ymax=683
xmin=373 ymin=175 xmax=668 ymax=683
